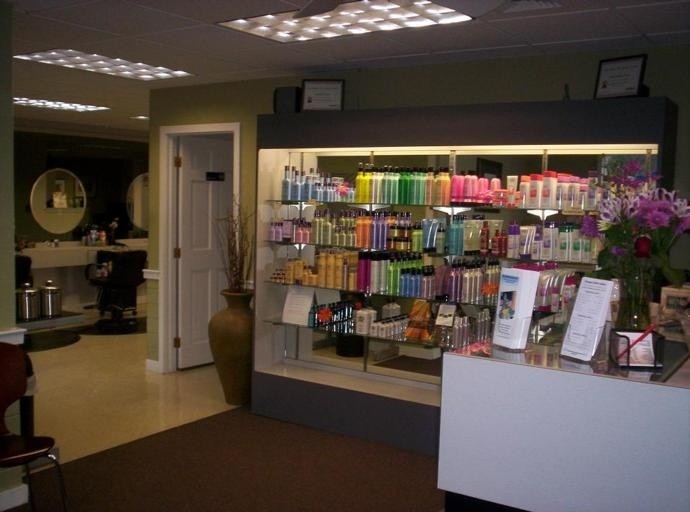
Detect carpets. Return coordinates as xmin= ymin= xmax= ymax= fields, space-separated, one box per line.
xmin=7 ymin=405 xmax=445 ymax=511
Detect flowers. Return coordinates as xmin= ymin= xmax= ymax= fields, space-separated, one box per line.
xmin=578 ymin=156 xmax=688 ymax=331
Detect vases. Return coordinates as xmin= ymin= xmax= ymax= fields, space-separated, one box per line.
xmin=207 ymin=285 xmax=256 ymax=406
xmin=615 ymin=274 xmax=653 ymax=330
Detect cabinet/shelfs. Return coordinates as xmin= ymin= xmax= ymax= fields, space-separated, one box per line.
xmin=250 ymin=98 xmax=682 ymax=459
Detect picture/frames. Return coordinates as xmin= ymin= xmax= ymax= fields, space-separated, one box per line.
xmin=592 ymin=52 xmax=649 ymax=99
xmin=299 ymin=80 xmax=346 ymax=114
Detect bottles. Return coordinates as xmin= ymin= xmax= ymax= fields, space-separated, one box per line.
xmin=17 ymin=280 xmax=64 ymax=322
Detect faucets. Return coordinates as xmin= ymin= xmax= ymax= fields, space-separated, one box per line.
xmin=53 ymin=239 xmax=59 ymax=248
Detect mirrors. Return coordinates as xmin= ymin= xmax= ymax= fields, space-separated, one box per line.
xmin=125 ymin=171 xmax=149 ymax=231
xmin=29 ymin=168 xmax=89 ymax=236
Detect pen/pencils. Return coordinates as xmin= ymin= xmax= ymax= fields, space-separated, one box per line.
xmin=614 ymin=323 xmax=655 ymax=362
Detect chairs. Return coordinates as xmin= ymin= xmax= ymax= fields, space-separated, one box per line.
xmin=83 ymin=249 xmax=149 ymax=335
xmin=0 ymin=344 xmax=71 ymax=510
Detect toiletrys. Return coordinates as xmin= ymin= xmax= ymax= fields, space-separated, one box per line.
xmin=268 ymin=161 xmax=620 ymax=352
